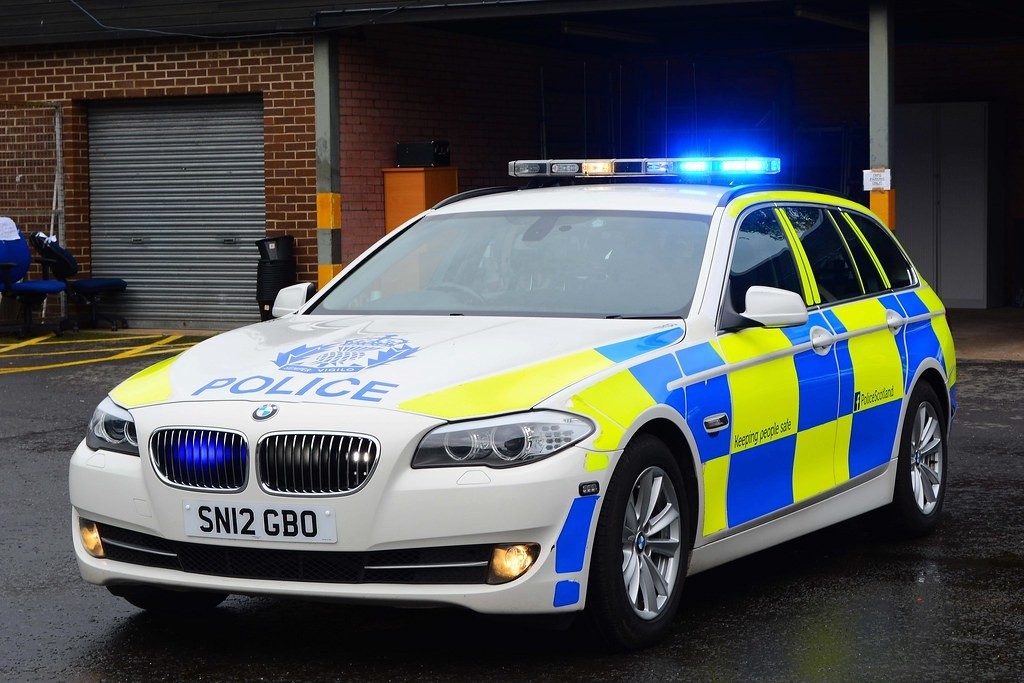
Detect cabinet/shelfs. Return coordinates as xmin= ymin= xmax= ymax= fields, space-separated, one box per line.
xmin=894 ymin=98 xmax=994 ymax=318
xmin=641 ymin=55 xmax=857 ymax=196
xmin=382 ymin=167 xmax=458 ymax=238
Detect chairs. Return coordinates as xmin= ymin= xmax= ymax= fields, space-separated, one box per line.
xmin=28 ymin=231 xmax=128 ymax=333
xmin=1 ymin=218 xmax=67 ymax=336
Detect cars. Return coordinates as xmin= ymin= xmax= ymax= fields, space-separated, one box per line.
xmin=68 ymin=157 xmax=959 ymax=652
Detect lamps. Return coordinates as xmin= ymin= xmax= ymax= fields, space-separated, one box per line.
xmin=562 ymin=21 xmax=657 ymax=46
xmin=795 ymin=7 xmax=870 ymax=33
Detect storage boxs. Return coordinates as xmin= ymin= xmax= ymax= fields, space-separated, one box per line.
xmin=255 ymin=234 xmax=296 ymax=322
